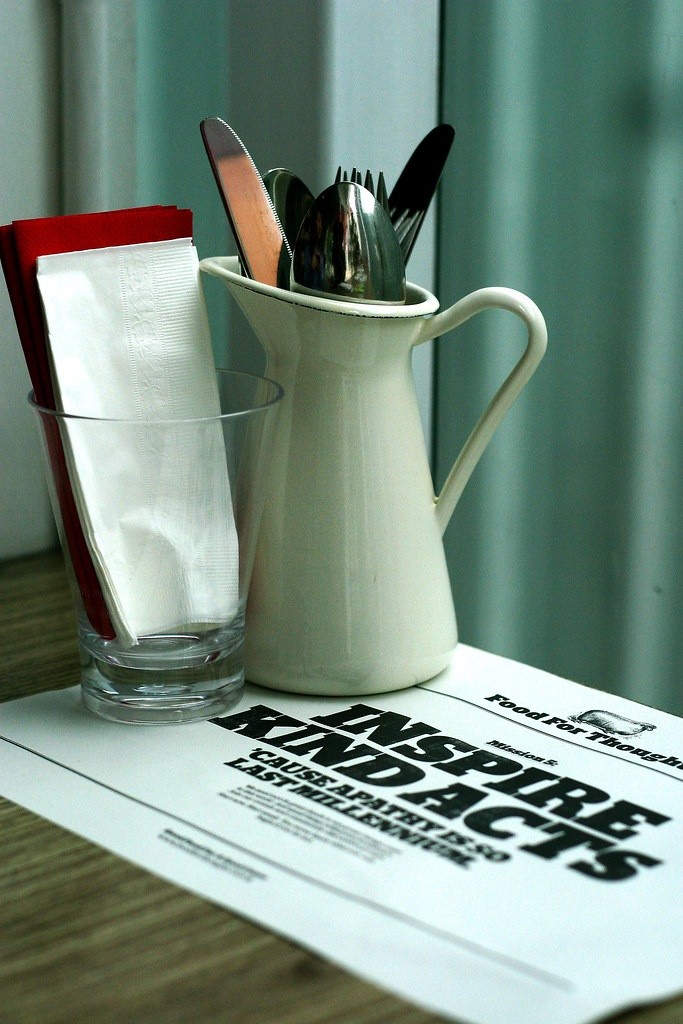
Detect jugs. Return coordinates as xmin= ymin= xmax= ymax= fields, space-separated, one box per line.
xmin=199 ymin=256 xmax=548 ymax=696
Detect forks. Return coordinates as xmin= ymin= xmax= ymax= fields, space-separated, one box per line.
xmin=334 ymin=166 xmax=424 ymax=263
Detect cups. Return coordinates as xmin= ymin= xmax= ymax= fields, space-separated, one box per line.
xmin=27 ymin=368 xmax=285 ymax=726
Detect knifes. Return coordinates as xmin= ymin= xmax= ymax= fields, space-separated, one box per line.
xmin=201 ymin=116 xmax=292 ymax=291
xmin=389 ymin=124 xmax=456 ymax=266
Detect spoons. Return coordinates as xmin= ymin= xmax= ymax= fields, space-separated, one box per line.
xmin=289 ymin=182 xmax=407 ymax=306
xmin=239 ymin=168 xmax=316 ymax=277
